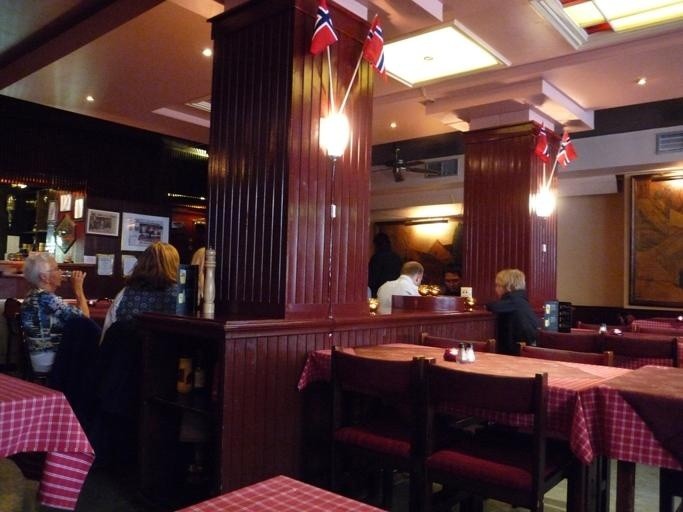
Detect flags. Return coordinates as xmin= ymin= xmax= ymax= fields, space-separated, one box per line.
xmin=534 ymin=123 xmax=551 ymax=163
xmin=309 ymin=0 xmax=339 ymax=56
xmin=362 ymin=15 xmax=387 ymax=83
xmin=556 ymin=131 xmax=578 ymax=167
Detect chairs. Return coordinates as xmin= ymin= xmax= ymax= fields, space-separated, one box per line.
xmin=1 ymin=293 xmax=36 ymax=379
xmin=390 ymin=292 xmax=681 ymax=370
xmin=327 ymin=345 xmax=425 ymax=509
xmin=421 ymin=357 xmax=570 ymax=510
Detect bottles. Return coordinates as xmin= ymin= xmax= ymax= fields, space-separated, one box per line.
xmin=599 ymin=321 xmax=608 ymax=333
xmin=460 ymin=343 xmax=476 ymax=363
xmin=177 ymin=346 xmax=205 ymax=394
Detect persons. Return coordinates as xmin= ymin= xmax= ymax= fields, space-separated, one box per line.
xmin=376 ymin=261 xmax=424 ymax=315
xmin=368 ymin=233 xmax=402 ymax=298
xmin=98 ymin=241 xmax=188 ymax=346
xmin=19 ymin=251 xmax=90 ymax=373
xmin=484 ymin=269 xmax=539 ymax=356
xmin=443 ymin=263 xmax=462 ymax=296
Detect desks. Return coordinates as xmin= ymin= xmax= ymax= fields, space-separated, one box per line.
xmin=595 ymin=364 xmax=682 ymax=511
xmin=296 ymin=343 xmax=637 ymax=510
xmin=2 ymin=372 xmax=97 ymax=510
xmin=66 ymin=298 xmax=113 ymax=320
xmin=176 ymin=473 xmax=385 ymax=510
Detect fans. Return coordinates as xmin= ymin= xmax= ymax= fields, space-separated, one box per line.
xmin=372 ymin=147 xmax=439 ymax=183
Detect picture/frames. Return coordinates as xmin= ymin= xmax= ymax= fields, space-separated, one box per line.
xmin=120 ymin=211 xmax=171 ymax=253
xmin=86 ymin=208 xmax=119 ymax=239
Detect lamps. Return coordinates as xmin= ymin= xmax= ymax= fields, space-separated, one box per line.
xmin=372 ymin=20 xmax=511 ymax=89
xmin=527 ymin=1 xmax=683 ymax=49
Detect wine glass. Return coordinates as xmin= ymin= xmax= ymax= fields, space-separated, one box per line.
xmin=61 ymin=270 xmax=87 ymax=278
